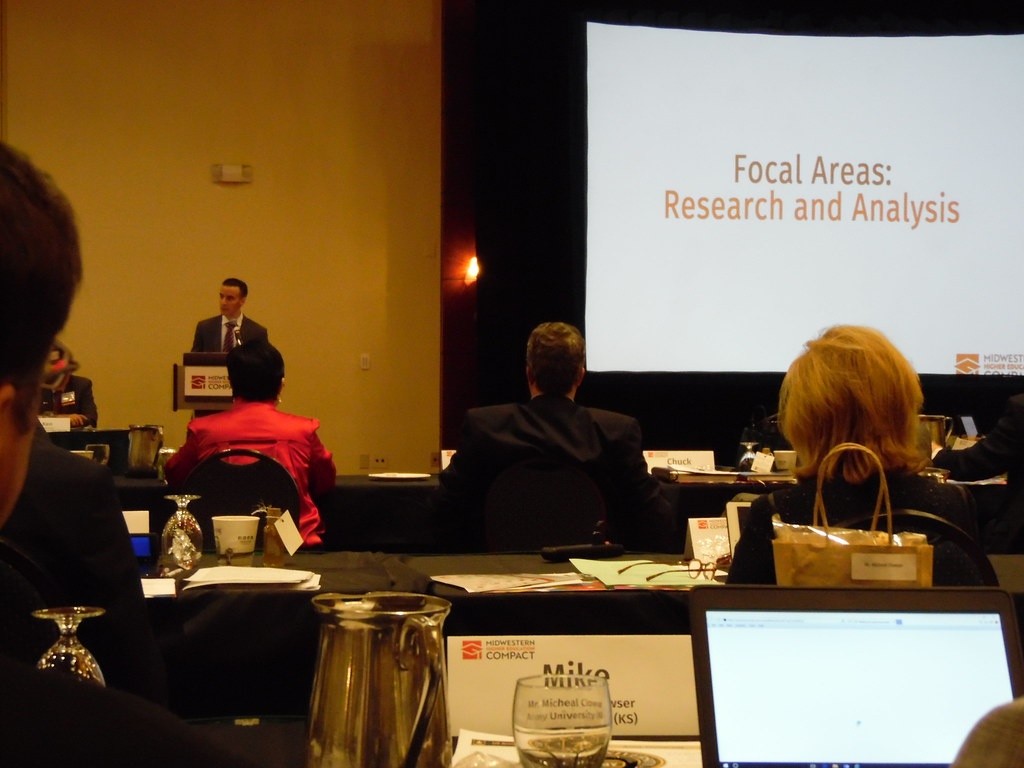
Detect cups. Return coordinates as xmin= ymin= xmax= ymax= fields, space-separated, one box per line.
xmin=918 ymin=467 xmax=950 ymax=482
xmin=512 ymin=676 xmax=611 ymax=768
xmin=774 ymin=451 xmax=797 ymax=469
xmin=159 ymin=448 xmax=178 ymax=482
xmin=86 ymin=445 xmax=110 ymax=464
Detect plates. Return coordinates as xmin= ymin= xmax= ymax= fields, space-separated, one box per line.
xmin=369 ymin=472 xmax=431 ymax=480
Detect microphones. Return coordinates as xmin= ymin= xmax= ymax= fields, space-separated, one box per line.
xmin=234 ymin=327 xmax=242 ymax=346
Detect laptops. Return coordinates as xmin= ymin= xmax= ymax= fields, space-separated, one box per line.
xmin=726 ymin=502 xmax=752 ymax=559
xmin=687 ymin=584 xmax=1024 ymax=768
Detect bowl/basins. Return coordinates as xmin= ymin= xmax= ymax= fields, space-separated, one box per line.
xmin=71 ymin=451 xmax=94 ymax=460
xmin=212 ymin=516 xmax=260 ymax=554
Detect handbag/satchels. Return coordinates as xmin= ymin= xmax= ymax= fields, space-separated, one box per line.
xmin=771 ymin=442 xmax=934 ymax=588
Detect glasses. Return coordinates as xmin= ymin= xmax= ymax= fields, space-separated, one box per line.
xmin=618 ymin=558 xmax=717 ymax=581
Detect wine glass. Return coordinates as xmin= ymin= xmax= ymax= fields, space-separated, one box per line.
xmin=740 ymin=443 xmax=759 ymax=472
xmin=33 ymin=607 xmax=107 ymax=688
xmin=162 ymin=496 xmax=203 ymax=568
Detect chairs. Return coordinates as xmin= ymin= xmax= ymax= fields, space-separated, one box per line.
xmin=834 ymin=509 xmax=1000 ymax=588
xmin=484 ymin=458 xmax=607 ymax=554
xmin=171 ymin=449 xmax=301 ymax=551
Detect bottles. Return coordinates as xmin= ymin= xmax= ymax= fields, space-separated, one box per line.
xmin=762 ymin=448 xmax=774 ymax=472
xmin=264 ymin=508 xmax=285 ymax=556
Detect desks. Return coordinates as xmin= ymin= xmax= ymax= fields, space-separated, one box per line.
xmin=101 ymin=462 xmax=1024 ymax=767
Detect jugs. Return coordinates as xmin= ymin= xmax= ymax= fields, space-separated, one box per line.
xmin=917 ymin=415 xmax=953 ymax=457
xmin=308 ymin=592 xmax=453 ymax=768
xmin=128 ymin=425 xmax=163 ymax=474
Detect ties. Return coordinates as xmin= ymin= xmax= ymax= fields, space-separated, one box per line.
xmin=222 ymin=321 xmax=237 ymax=353
xmin=52 ymin=390 xmax=62 ymax=409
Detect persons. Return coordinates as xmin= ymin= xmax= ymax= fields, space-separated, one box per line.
xmin=189 ymin=278 xmax=270 ymax=355
xmin=0 ymin=136 xmax=264 ymax=766
xmin=163 ymin=342 xmax=337 ymax=554
xmin=724 ymin=328 xmax=1001 ymax=587
xmin=932 ymin=395 xmax=1024 ymax=557
xmin=37 ymin=375 xmax=97 ymax=431
xmin=438 ymin=321 xmax=676 ymax=554
